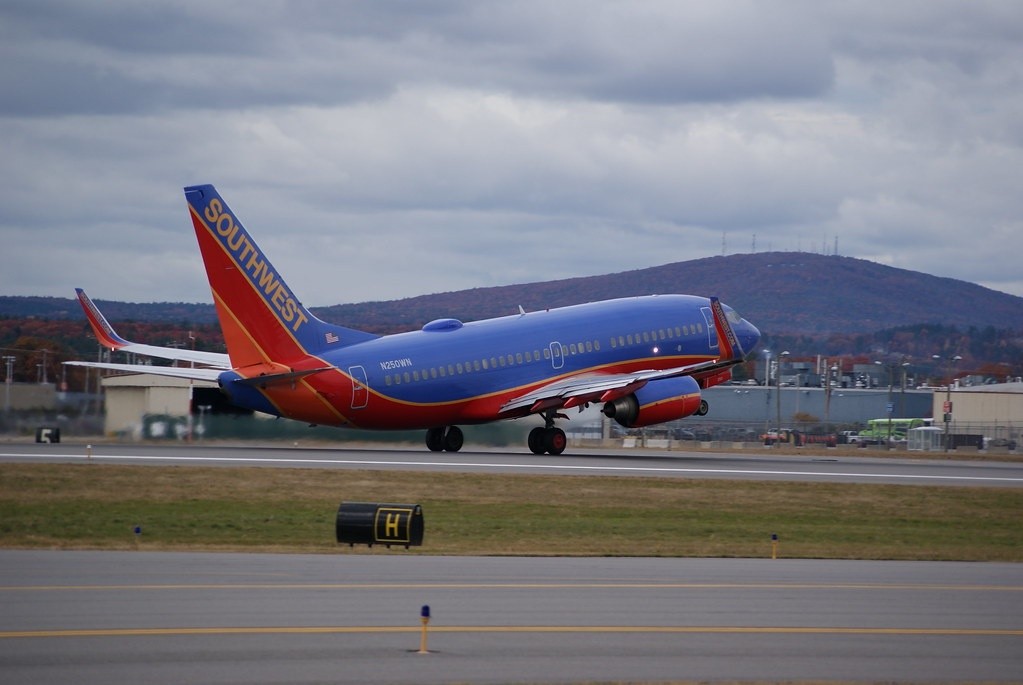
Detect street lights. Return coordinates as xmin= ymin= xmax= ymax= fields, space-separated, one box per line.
xmin=764 ymin=350 xmax=792 ymax=447
xmin=874 ymin=359 xmax=910 ymax=451
xmin=930 ymin=354 xmax=963 ymax=453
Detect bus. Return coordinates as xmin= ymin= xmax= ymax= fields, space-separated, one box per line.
xmin=858 ymin=418 xmax=926 ymax=443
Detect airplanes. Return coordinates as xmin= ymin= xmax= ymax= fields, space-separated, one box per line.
xmin=54 ymin=182 xmax=768 ymax=460
